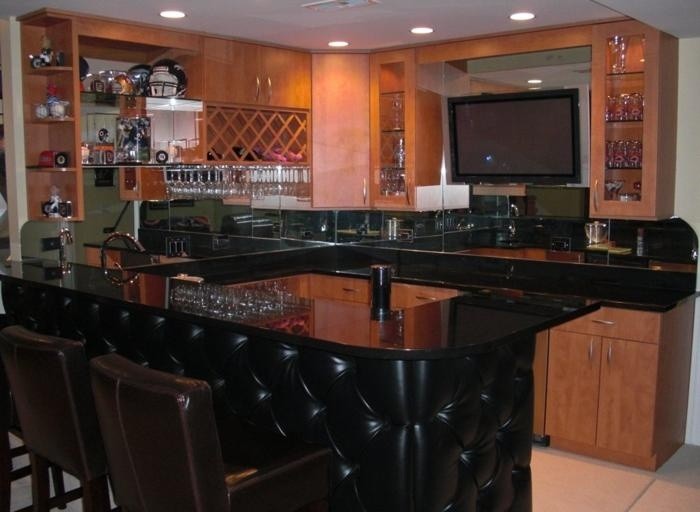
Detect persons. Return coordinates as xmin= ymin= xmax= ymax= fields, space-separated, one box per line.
xmin=47 ymin=184 xmax=62 ymax=215
xmin=39 ymin=32 xmax=52 ymax=64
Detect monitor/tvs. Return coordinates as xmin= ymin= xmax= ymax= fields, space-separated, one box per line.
xmin=448 ymin=88 xmax=581 ymax=183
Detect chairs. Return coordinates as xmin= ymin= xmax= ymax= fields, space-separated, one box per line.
xmin=91 ymin=353 xmax=329 ymax=512
xmin=0 ymin=326 xmax=111 ymax=512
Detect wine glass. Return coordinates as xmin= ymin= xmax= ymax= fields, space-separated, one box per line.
xmin=168 ymin=166 xmax=311 ymax=200
xmin=173 ymin=283 xmax=301 ymax=324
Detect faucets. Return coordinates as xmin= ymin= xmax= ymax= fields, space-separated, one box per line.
xmin=100 ymin=232 xmax=146 ymax=270
xmin=57 ymin=227 xmax=75 ymax=268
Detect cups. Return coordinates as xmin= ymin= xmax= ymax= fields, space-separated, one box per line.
xmin=380 ymin=169 xmax=406 ymax=197
xmin=606 ymin=175 xmax=641 ymax=200
xmin=607 ymin=93 xmax=641 ymax=121
xmin=607 ymin=141 xmax=642 ymax=169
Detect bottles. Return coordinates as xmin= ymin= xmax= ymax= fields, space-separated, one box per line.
xmin=391 ymin=95 xmax=404 ymax=130
xmin=609 ymin=37 xmax=626 ymax=73
xmin=393 ymin=136 xmax=406 ymax=167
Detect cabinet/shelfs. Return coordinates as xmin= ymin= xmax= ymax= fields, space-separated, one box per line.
xmin=310 ymin=275 xmax=374 ymax=305
xmin=534 ymin=329 xmax=548 ymax=446
xmin=202 ymin=36 xmax=311 ymax=111
xmin=224 ymin=274 xmax=313 ymax=337
xmin=15 ymin=7 xmax=84 ymax=223
xmin=72 ymin=10 xmax=203 ymax=169
xmin=589 ymin=14 xmax=679 ymax=221
xmin=370 ymin=43 xmax=444 ymax=213
xmin=544 ymin=297 xmax=695 ymax=473
xmin=392 ymin=282 xmax=470 ymax=311
xmin=312 ymin=51 xmax=369 ymax=211
